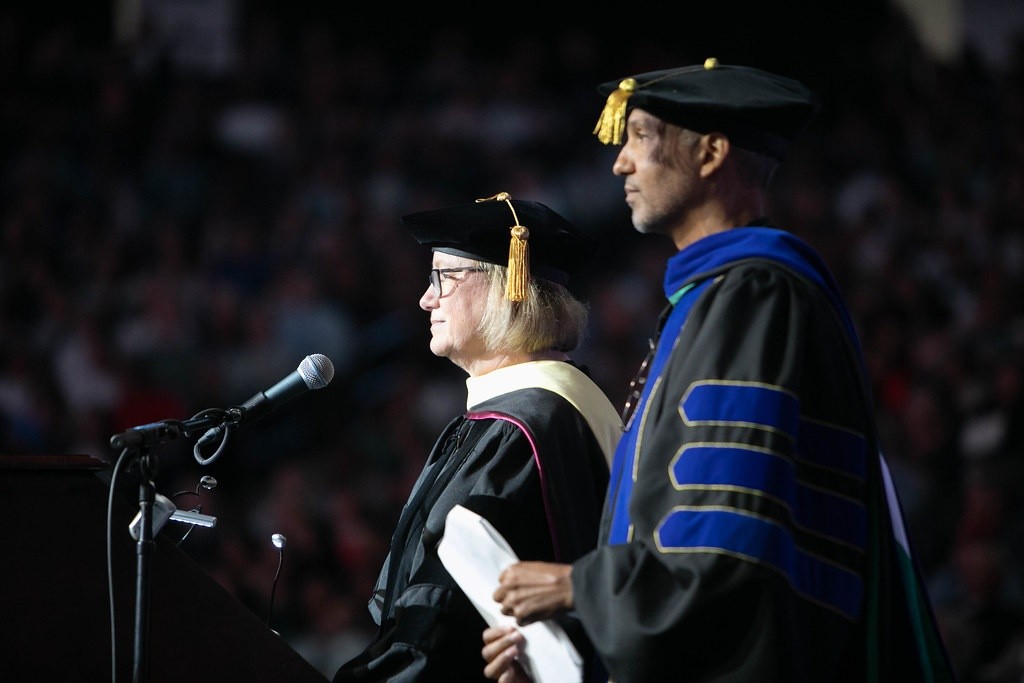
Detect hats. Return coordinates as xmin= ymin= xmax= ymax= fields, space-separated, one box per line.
xmin=400 ymin=193 xmax=585 ymax=302
xmin=591 ymin=58 xmax=811 ymax=163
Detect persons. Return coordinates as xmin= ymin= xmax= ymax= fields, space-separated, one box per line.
xmin=480 ymin=56 xmax=959 ymax=683
xmin=332 ymin=192 xmax=624 ymax=683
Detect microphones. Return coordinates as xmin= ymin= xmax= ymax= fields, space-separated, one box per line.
xmin=198 ymin=352 xmax=335 ymax=448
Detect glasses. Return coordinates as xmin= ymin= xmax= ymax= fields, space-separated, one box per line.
xmin=428 ymin=267 xmax=493 ymax=298
